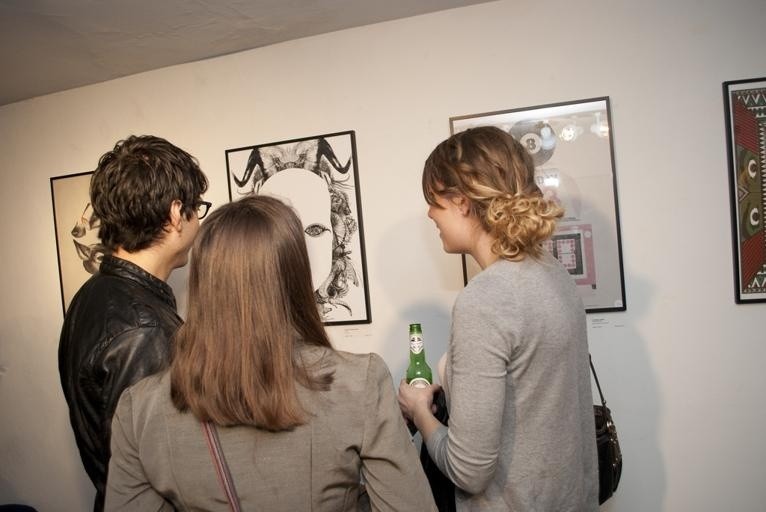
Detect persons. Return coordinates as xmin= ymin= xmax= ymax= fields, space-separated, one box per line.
xmin=396 ymin=125 xmax=604 ymax=511
xmin=55 ymin=133 xmax=209 ymax=512
xmin=103 ymin=193 xmax=441 ymax=512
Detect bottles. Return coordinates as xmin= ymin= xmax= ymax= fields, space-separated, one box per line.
xmin=406 ymin=324 xmax=432 ymax=389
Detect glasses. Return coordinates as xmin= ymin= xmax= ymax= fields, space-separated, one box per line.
xmin=181 ymin=197 xmax=213 ymax=220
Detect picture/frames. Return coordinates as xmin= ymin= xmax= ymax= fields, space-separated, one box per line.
xmin=447 ymin=96 xmax=627 ymax=314
xmin=720 ymin=77 xmax=766 ymax=304
xmin=225 ymin=131 xmax=371 ymax=326
xmin=48 ymin=171 xmax=104 ymax=318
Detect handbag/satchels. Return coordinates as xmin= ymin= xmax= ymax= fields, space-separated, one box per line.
xmin=417 ymin=353 xmax=623 ymax=512
